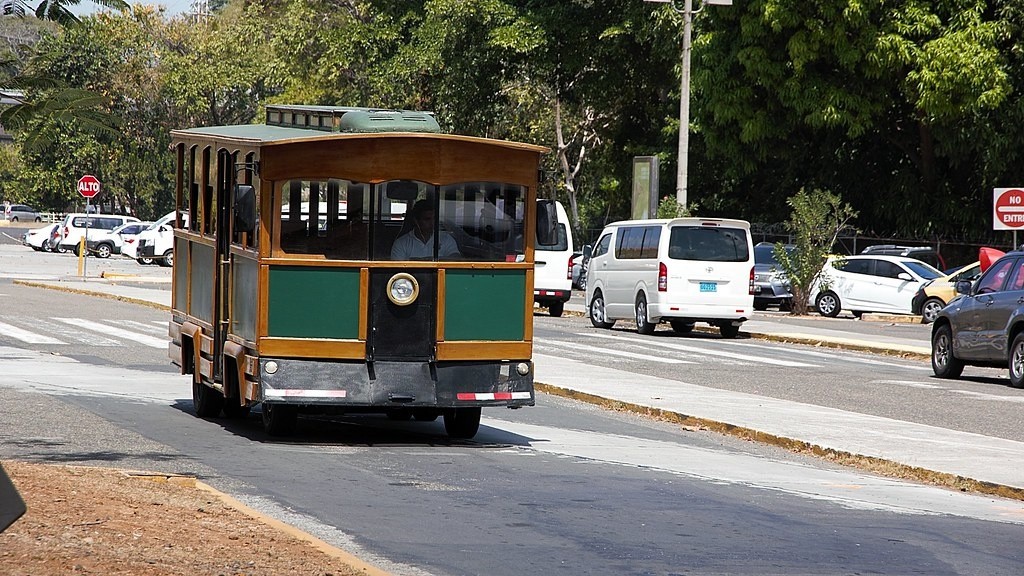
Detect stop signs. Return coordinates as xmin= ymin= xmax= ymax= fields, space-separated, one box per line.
xmin=992 ymin=187 xmax=1024 ymax=229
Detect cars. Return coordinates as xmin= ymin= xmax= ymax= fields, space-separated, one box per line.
xmin=912 ymin=262 xmax=986 ymax=323
xmin=22 ymin=209 xmax=190 ymax=267
xmin=0 ymin=204 xmax=43 ymax=223
xmin=805 ymin=254 xmax=949 ymax=318
xmin=929 ymin=244 xmax=1024 ymax=389
xmin=572 ymin=251 xmax=587 ymax=292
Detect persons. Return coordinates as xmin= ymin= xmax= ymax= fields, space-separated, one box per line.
xmin=391 ymin=199 xmax=460 ymax=261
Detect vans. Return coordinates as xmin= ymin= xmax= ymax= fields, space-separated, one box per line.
xmin=582 ymin=218 xmax=757 ymax=337
xmin=516 ymin=199 xmax=575 ymax=317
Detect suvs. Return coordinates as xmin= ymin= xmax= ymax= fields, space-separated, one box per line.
xmin=751 ymin=240 xmax=806 ymax=311
xmin=853 ymin=245 xmax=948 ymax=274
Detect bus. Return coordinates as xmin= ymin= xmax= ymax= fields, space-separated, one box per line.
xmin=167 ymin=101 xmax=542 ymax=442
